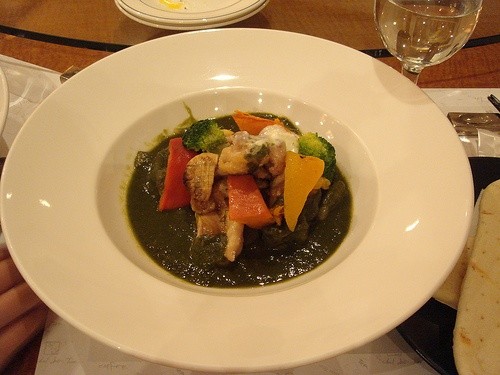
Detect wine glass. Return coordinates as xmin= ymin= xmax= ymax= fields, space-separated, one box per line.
xmin=373 ymin=0 xmax=482 ymax=86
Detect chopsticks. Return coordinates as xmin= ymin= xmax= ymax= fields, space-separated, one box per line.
xmin=488 ymin=94 xmax=500 ymax=112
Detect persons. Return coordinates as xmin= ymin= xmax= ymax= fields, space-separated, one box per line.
xmin=0 ymin=244 xmax=47 ymax=375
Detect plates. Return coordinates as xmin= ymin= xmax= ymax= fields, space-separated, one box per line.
xmin=0 ymin=68 xmax=9 ymax=136
xmin=0 ymin=29 xmax=475 ymax=372
xmin=392 ymin=156 xmax=500 ymax=375
xmin=113 ymin=0 xmax=271 ymax=31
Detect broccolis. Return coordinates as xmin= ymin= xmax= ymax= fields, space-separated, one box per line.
xmin=180 ymin=118 xmax=227 ymax=152
xmin=297 ymin=132 xmax=336 ymax=174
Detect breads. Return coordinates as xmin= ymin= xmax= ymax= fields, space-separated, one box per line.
xmin=432 ymin=178 xmax=500 ymax=375
